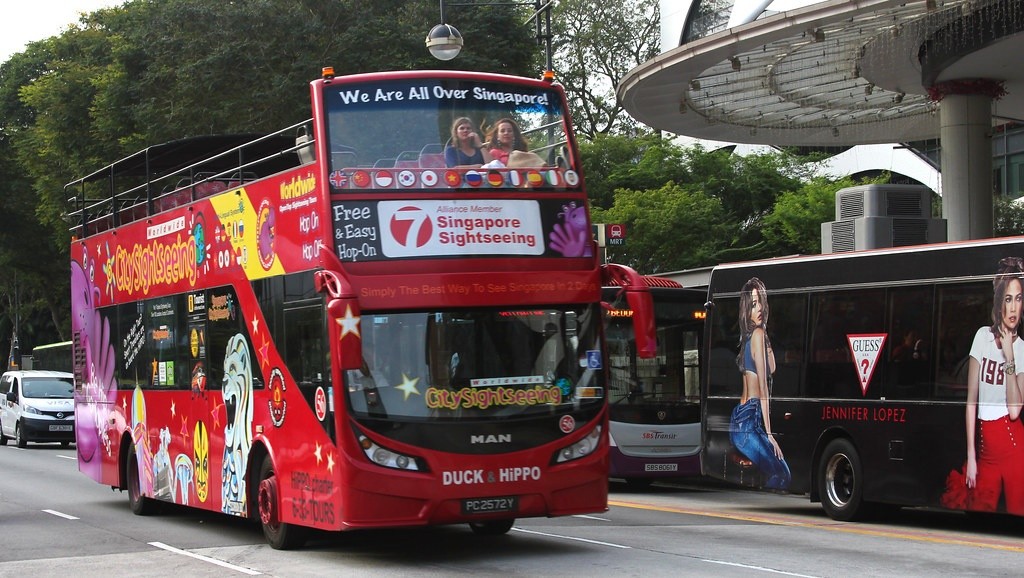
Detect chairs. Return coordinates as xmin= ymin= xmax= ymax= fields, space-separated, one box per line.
xmin=784 ymin=345 xmax=853 ymax=363
xmin=352 ymin=136 xmax=455 ymax=190
xmin=72 ymin=173 xmax=259 ymax=242
xmin=640 ymin=377 xmax=683 ymax=406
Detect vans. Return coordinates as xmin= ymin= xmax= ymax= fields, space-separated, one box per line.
xmin=0 ymin=370 xmax=76 ymax=448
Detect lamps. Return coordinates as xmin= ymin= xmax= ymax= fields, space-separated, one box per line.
xmin=680 ymin=0 xmax=972 ymax=136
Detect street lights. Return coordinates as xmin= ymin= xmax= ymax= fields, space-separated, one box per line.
xmin=425 ymin=0 xmax=557 ymax=168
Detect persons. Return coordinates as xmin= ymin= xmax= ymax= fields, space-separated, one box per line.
xmin=728 ymin=277 xmax=795 ymax=490
xmin=965 ymin=257 xmax=1024 ymax=516
xmin=445 ymin=116 xmax=497 ymax=169
xmin=480 ymin=118 xmax=528 ymax=168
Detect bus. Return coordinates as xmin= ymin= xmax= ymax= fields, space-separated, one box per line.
xmin=699 ymin=235 xmax=1024 ymax=522
xmin=63 ymin=65 xmax=656 ymax=552
xmin=603 ymin=276 xmax=707 ymax=490
